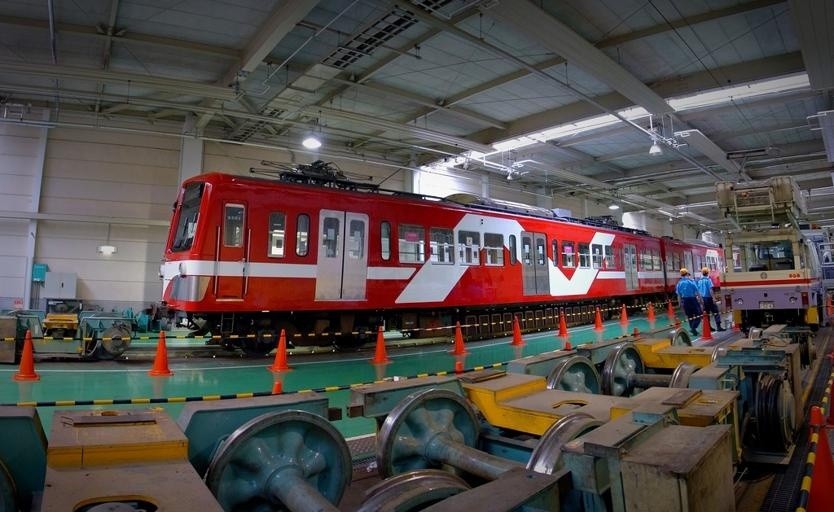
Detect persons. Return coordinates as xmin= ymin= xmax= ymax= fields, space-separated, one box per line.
xmin=675 ymin=268 xmax=705 ymax=336
xmin=708 ymin=268 xmax=722 ymax=305
xmin=698 ymin=267 xmax=727 ymax=332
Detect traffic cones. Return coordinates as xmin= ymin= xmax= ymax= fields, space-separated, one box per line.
xmin=808 ymin=352 xmax=834 ymax=512
xmin=700 ymin=311 xmax=714 ymax=340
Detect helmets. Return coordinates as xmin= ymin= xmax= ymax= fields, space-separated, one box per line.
xmin=679 ymin=268 xmax=688 ymax=276
xmin=701 ymin=267 xmax=709 ymax=275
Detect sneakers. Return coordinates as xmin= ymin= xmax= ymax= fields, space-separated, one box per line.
xmin=711 ymin=328 xmax=715 ymax=331
xmin=716 ymin=328 xmax=726 ymax=331
xmin=691 ymin=329 xmax=698 ymax=336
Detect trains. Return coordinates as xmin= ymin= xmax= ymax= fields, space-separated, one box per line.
xmin=156 ymin=158 xmax=726 ymax=353
xmin=715 ymin=174 xmax=826 ymax=335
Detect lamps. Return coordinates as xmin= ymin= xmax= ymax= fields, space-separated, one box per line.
xmin=647 ymin=114 xmax=663 ymax=155
